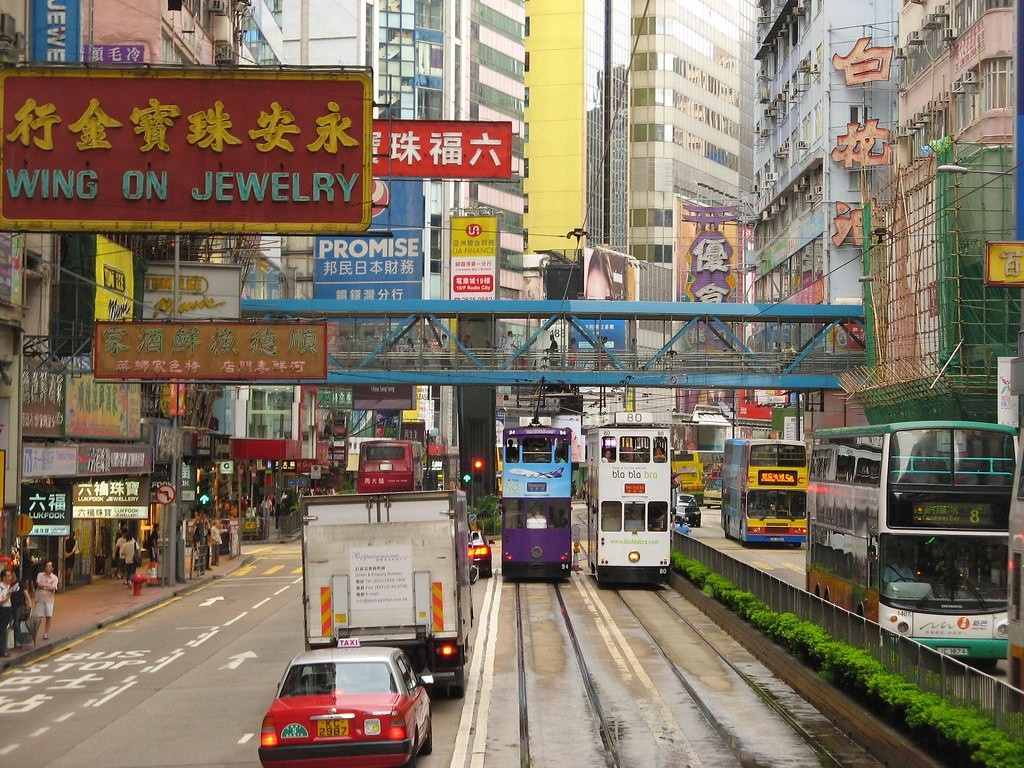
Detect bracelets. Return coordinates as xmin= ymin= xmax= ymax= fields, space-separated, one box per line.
xmin=44 ymin=587 xmax=46 ymax=590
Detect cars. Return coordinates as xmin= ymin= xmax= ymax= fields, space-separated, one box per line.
xmin=674 ymin=495 xmax=700 ymax=527
xmin=468 ymin=530 xmax=495 ymax=578
xmin=257 ymin=636 xmax=435 ymax=768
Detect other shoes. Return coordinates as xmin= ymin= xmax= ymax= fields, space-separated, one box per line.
xmin=2 ymin=652 xmax=9 ymax=657
xmin=43 ymin=634 xmax=48 ymax=640
xmin=128 ymin=580 xmax=132 ymax=590
xmin=16 ymin=642 xmax=22 ymax=648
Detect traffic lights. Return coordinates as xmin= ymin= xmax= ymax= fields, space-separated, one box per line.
xmin=197 ymin=475 xmax=210 ymax=507
xmin=463 ymin=458 xmax=483 ymax=484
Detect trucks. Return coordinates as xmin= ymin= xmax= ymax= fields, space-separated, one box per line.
xmin=703 ymin=477 xmax=723 ymax=509
xmin=300 ymin=489 xmax=480 ymax=701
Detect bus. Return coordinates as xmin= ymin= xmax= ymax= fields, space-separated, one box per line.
xmin=674 ymin=451 xmax=724 ymax=492
xmin=358 ymin=440 xmax=424 ymax=494
xmin=720 ymin=438 xmax=808 ymax=549
xmin=803 ymin=419 xmax=1017 ymax=676
xmin=496 ymin=446 xmax=503 ymax=491
xmin=586 ymin=428 xmax=673 ymax=586
xmin=1007 ymin=428 xmax=1024 ymax=712
xmin=502 ymin=428 xmax=572 ymax=578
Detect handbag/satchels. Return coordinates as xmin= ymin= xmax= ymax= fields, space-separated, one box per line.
xmin=133 ymin=552 xmax=142 ymax=567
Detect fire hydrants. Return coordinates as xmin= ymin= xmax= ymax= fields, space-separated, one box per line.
xmin=132 ymin=575 xmax=146 ymax=597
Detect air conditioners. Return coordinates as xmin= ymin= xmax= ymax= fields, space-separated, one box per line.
xmin=216 ymin=44 xmax=234 ymax=65
xmin=744 ymin=7 xmax=822 ymax=351
xmin=209 ymin=0 xmax=226 ymax=13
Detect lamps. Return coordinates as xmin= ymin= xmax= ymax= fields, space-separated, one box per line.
xmin=936 ymin=165 xmax=1011 ymax=179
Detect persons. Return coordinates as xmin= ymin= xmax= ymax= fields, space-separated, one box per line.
xmin=0 ymin=569 xmax=32 ymax=658
xmin=381 ymin=333 xmax=640 ymax=371
xmin=760 ymin=498 xmax=786 ymax=519
xmin=774 ymin=342 xmax=798 ymax=374
xmin=22 ymin=535 xmax=36 ymax=593
xmin=193 ymin=483 xmax=338 ymax=571
xmin=64 ymin=531 xmax=78 ymax=586
xmin=508 ymin=438 xmax=566 ymax=463
xmin=33 ymin=560 xmax=58 ymax=640
xmin=571 ymin=480 xmax=591 ymax=502
xmin=884 ymin=555 xmax=916 ymax=585
xmin=517 ymin=503 xmax=568 ymax=531
xmin=586 ymin=249 xmax=616 ymax=301
xmin=602 ymin=446 xmax=693 ymax=535
xmin=111 ymin=523 xmax=141 ymax=588
xmin=148 ymin=524 xmax=159 ymax=562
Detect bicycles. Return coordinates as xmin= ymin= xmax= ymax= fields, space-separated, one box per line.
xmin=571 ymin=489 xmax=584 ymax=501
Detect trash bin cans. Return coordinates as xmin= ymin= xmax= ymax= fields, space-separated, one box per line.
xmin=18 ymin=612 xmax=33 ymax=644
xmin=219 ymin=531 xmax=230 ymax=555
